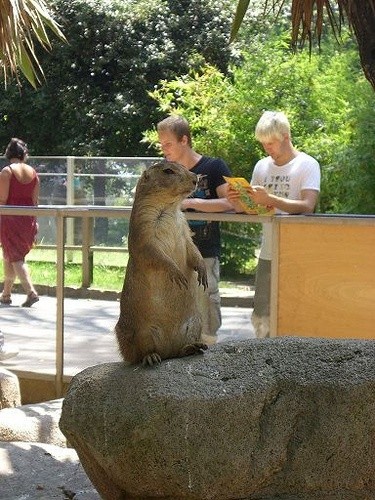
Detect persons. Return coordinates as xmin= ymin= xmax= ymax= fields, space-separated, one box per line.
xmin=156 ymin=115 xmax=235 ymax=344
xmin=225 ymin=110 xmax=322 ymax=340
xmin=0 ymin=137 xmax=40 ymax=307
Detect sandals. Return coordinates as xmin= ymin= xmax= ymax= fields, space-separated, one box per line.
xmin=22 ymin=291 xmax=40 ymax=307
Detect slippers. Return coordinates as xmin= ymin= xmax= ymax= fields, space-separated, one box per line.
xmin=0 ymin=290 xmax=12 ymax=305
